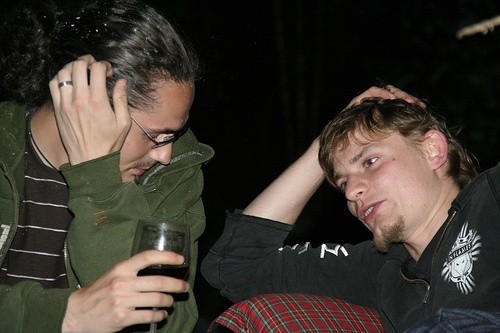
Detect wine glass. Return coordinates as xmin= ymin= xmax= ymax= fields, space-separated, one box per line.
xmin=130 ymin=219 xmax=192 ymax=333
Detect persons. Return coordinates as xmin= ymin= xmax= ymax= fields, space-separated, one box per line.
xmin=0 ymin=0 xmax=214 ymax=333
xmin=200 ymin=85 xmax=499 ymax=332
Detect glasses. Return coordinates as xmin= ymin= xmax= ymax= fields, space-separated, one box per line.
xmin=128 ymin=114 xmax=189 ymax=148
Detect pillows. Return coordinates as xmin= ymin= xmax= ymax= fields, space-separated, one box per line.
xmin=207 ymin=293 xmax=385 ymax=333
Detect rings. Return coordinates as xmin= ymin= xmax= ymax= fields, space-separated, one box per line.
xmin=59 ymin=81 xmax=73 ymax=88
